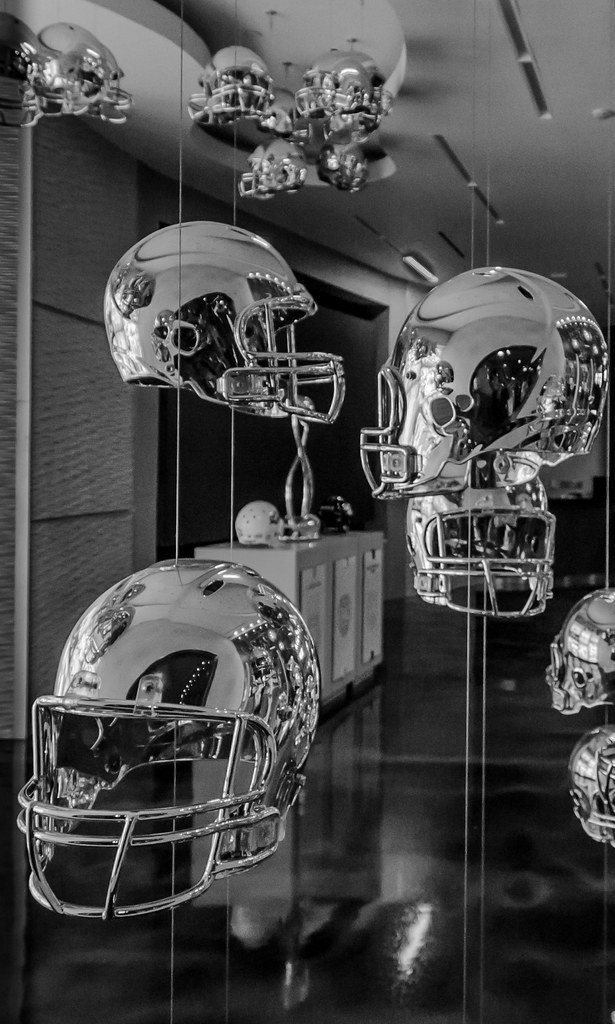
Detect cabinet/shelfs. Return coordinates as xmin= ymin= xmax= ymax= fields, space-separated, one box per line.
xmin=192 ymin=530 xmax=386 ymax=716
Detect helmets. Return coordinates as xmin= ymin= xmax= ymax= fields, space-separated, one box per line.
xmin=317 ymin=496 xmax=352 ymax=534
xmin=360 ymin=266 xmax=611 ymax=499
xmin=236 ymin=501 xmax=284 ymax=549
xmin=15 ymin=24 xmax=133 ymax=128
xmin=545 ymin=589 xmax=615 ymax=715
xmin=17 ymin=560 xmax=319 ymax=920
xmin=405 ymin=475 xmax=554 ymax=618
xmin=568 ymin=725 xmax=615 ymax=848
xmin=186 ymin=47 xmax=394 ymax=199
xmin=103 ymin=221 xmax=345 ymax=423
